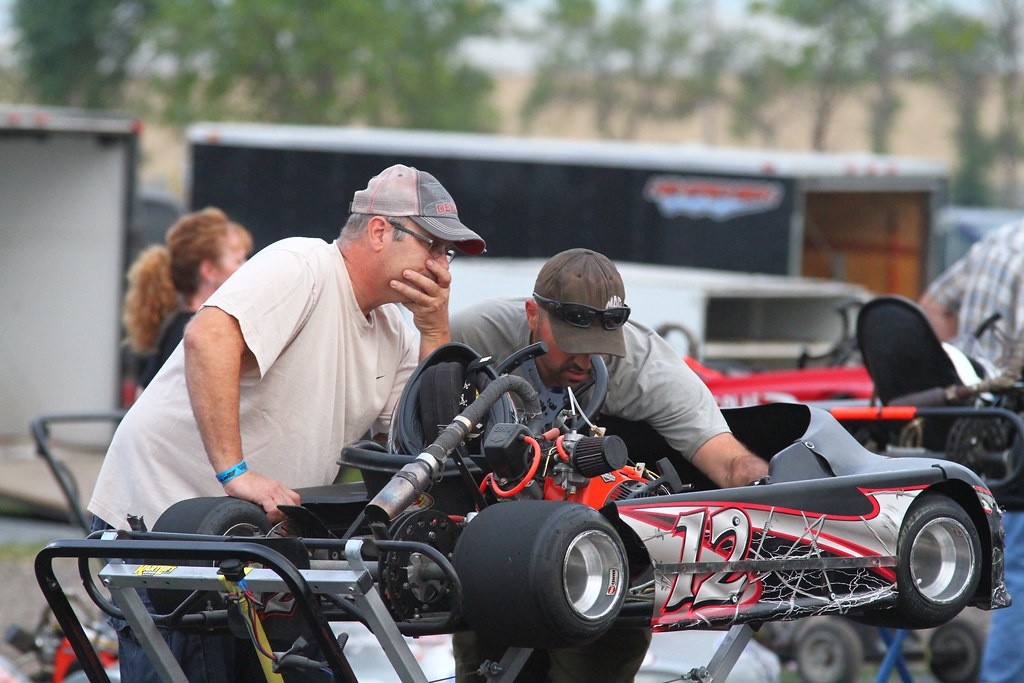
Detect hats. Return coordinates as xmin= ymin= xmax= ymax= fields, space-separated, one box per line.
xmin=350 ymin=162 xmax=486 ymax=255
xmin=533 ymin=247 xmax=628 ymax=357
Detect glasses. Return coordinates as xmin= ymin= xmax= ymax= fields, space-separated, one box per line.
xmin=385 ymin=217 xmax=454 ymax=264
xmin=533 ymin=291 xmax=630 ymax=330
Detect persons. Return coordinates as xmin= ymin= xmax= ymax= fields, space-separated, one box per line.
xmin=445 ymin=246 xmax=771 ymax=682
xmin=914 ymin=215 xmax=1024 ymax=683
xmin=88 ymin=163 xmax=487 ymax=682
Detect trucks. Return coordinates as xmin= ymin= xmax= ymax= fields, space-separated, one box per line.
xmin=185 ymin=121 xmax=955 ymax=375
xmin=0 ymin=99 xmax=143 ymax=453
